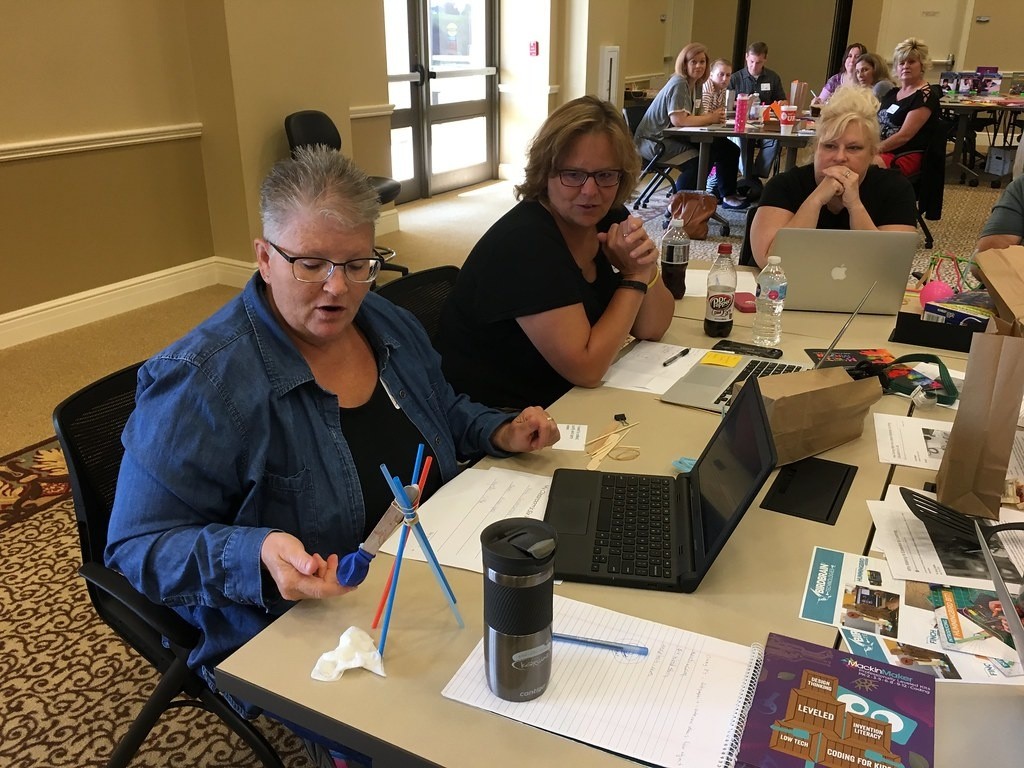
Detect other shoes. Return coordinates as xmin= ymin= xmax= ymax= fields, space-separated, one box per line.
xmin=736 ymin=178 xmax=751 ymax=197
xmin=746 ymin=176 xmax=763 ymax=201
xmin=722 ymin=200 xmax=751 ymax=209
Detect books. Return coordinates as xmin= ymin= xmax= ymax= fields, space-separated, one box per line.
xmin=940 ymin=66 xmax=1002 ymax=95
xmin=441 ymin=593 xmax=763 ymax=768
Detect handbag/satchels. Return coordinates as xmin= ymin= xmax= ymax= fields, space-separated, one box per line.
xmin=671 ymin=192 xmax=718 ymax=240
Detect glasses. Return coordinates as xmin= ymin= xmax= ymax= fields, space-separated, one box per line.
xmin=554 ymin=166 xmax=624 ymax=187
xmin=266 ymin=240 xmax=384 ymax=283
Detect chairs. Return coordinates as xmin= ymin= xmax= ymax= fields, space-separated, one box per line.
xmin=371 ymin=265 xmax=460 ymax=349
xmin=890 ymin=113 xmax=958 ymax=250
xmin=51 ymin=359 xmax=287 ymax=768
xmin=623 ymin=105 xmax=677 ymax=210
xmin=284 ymin=110 xmax=409 ymax=290
xmin=738 ymin=207 xmax=933 ymax=286
xmin=931 ymin=84 xmax=1024 ymax=188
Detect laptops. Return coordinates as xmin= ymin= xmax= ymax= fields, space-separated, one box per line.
xmin=772 ymin=227 xmax=920 ymax=314
xmin=660 ymin=280 xmax=879 ymax=412
xmin=543 ymin=373 xmax=779 ymax=594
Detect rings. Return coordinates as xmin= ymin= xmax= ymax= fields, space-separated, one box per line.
xmin=547 ymin=418 xmax=552 ymax=420
xmin=844 ymin=171 xmax=851 ymax=177
xmin=623 ymin=234 xmax=628 ymax=236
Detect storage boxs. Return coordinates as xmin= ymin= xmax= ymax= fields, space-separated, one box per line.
xmin=984 ymin=146 xmax=1017 ymax=177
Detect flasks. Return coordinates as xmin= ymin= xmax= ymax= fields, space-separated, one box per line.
xmin=480 ymin=517 xmax=557 ymax=703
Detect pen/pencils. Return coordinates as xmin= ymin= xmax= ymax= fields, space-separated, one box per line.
xmin=551 ymin=630 xmax=649 ymax=661
xmin=664 ymin=348 xmax=692 ymax=367
xmin=811 ymin=89 xmax=816 ymax=98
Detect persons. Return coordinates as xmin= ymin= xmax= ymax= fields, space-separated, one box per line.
xmin=738 ymin=84 xmax=917 ymax=272
xmin=810 ymin=43 xmax=867 ymax=105
xmin=727 ymin=42 xmax=788 ymax=190
xmin=941 ymin=79 xmax=951 ymax=92
xmin=434 ymin=92 xmax=676 ymax=408
xmin=698 ymin=57 xmax=751 ymax=209
xmin=981 ymin=79 xmax=992 ymax=91
xmin=855 ymin=52 xmax=897 ymax=100
xmin=104 ymin=145 xmax=561 ymax=768
xmin=971 ymin=174 xmax=1024 ymax=282
xmin=633 ymin=43 xmax=727 ymax=193
xmin=870 ymin=37 xmax=941 ymax=176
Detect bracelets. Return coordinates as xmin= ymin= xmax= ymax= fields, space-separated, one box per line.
xmin=617 ymin=280 xmax=648 ymax=294
xmin=648 ymin=266 xmax=660 ymax=289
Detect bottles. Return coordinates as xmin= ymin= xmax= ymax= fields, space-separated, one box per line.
xmin=735 ymin=93 xmax=748 ymax=132
xmin=703 ymin=244 xmax=737 ymax=337
xmin=751 ymin=255 xmax=787 ymax=348
xmin=660 ymin=217 xmax=691 ymax=300
xmin=750 ymin=93 xmax=760 ymax=120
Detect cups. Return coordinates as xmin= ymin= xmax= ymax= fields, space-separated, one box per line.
xmin=725 ymin=90 xmax=735 ymax=111
xmin=779 ymin=105 xmax=797 ymax=135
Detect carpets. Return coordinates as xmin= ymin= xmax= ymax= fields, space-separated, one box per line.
xmin=624 ymin=150 xmax=1024 ymax=349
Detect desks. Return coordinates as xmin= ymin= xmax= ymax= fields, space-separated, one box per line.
xmin=213 ymin=261 xmax=1024 ymax=768
xmin=663 ymin=110 xmax=820 ymax=236
xmin=939 ymin=93 xmax=1024 ymax=178
